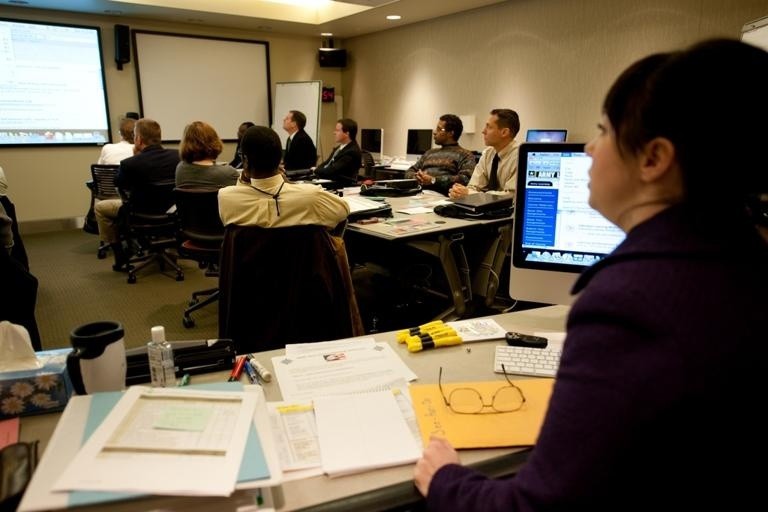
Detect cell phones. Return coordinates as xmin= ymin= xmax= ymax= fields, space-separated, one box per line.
xmin=506 ymin=331 xmax=547 ymax=347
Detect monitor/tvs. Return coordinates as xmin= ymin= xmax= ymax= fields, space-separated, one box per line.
xmin=361 ymin=128 xmax=383 ymax=163
xmin=406 ymin=128 xmax=433 ymax=161
xmin=526 ymin=129 xmax=568 ymax=143
xmin=509 ymin=143 xmax=627 ymax=305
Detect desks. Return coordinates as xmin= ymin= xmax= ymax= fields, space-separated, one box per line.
xmin=1 ymin=301 xmax=572 ymax=511
xmin=341 ymin=178 xmax=515 ymax=327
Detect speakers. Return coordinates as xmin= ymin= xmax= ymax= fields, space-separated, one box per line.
xmin=114 ymin=24 xmax=130 ymax=64
xmin=319 ymin=48 xmax=348 ymax=67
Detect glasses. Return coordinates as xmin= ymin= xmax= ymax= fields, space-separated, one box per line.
xmin=439 ymin=364 xmax=525 ymax=414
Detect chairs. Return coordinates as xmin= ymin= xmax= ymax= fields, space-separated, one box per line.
xmin=90 ymin=161 xmax=119 ymax=261
xmin=173 ymin=186 xmax=223 ymax=325
xmin=217 ymin=221 xmax=362 ymax=352
xmin=115 ymin=185 xmax=186 ymax=284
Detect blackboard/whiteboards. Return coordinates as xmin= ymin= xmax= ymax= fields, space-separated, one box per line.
xmin=273 ymin=80 xmax=322 ymax=152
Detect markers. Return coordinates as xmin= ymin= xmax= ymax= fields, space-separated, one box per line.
xmin=228 ymin=354 xmax=273 ymax=385
xmin=396 ymin=320 xmax=462 ymax=352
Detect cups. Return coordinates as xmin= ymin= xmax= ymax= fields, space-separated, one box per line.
xmin=66 ymin=319 xmax=128 ymax=395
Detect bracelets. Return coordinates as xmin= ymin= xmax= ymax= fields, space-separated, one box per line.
xmin=431 ymin=177 xmax=435 ymax=184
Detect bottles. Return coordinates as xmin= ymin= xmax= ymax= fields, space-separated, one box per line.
xmin=145 ymin=325 xmax=176 ymax=387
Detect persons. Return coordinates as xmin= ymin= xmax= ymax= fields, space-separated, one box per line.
xmin=278 ymin=110 xmax=317 ymax=171
xmin=218 ymin=126 xmax=350 ymax=228
xmin=98 ymin=118 xmax=135 ymax=165
xmin=405 ymin=114 xmax=476 ymax=199
xmin=229 ymin=122 xmax=255 ymax=169
xmin=311 ymin=118 xmax=362 ymax=187
xmin=175 ymin=122 xmax=240 ymax=187
xmin=439 ymin=109 xmax=520 ymax=280
xmin=0 ymin=163 xmax=31 ymax=270
xmin=96 ymin=118 xmax=180 ymax=268
xmin=414 ymin=38 xmax=767 ymax=510
xmin=0 ymin=191 xmax=42 ymax=351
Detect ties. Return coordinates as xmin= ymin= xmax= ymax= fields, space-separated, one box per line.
xmin=489 ymin=153 xmax=501 ymax=190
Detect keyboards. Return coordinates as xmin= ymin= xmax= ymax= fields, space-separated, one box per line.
xmin=493 ymin=344 xmax=562 ymax=379
